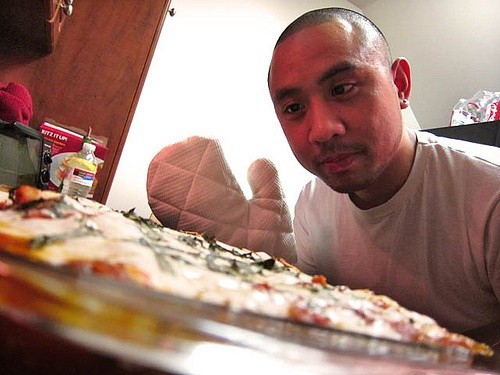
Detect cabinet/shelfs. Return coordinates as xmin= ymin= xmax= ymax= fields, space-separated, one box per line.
xmin=13 ymin=0 xmax=73 ymax=55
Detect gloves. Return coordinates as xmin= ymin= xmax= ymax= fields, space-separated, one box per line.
xmin=146 ymin=136 xmax=299 ymax=267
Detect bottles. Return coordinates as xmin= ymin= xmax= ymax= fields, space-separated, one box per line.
xmin=61 ymin=143 xmax=97 ymax=198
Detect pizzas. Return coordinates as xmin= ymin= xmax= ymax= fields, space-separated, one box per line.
xmin=0 ymin=183 xmax=495 ymax=357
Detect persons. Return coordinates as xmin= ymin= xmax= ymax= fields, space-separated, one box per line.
xmin=146 ymin=7 xmax=496 ymax=375
